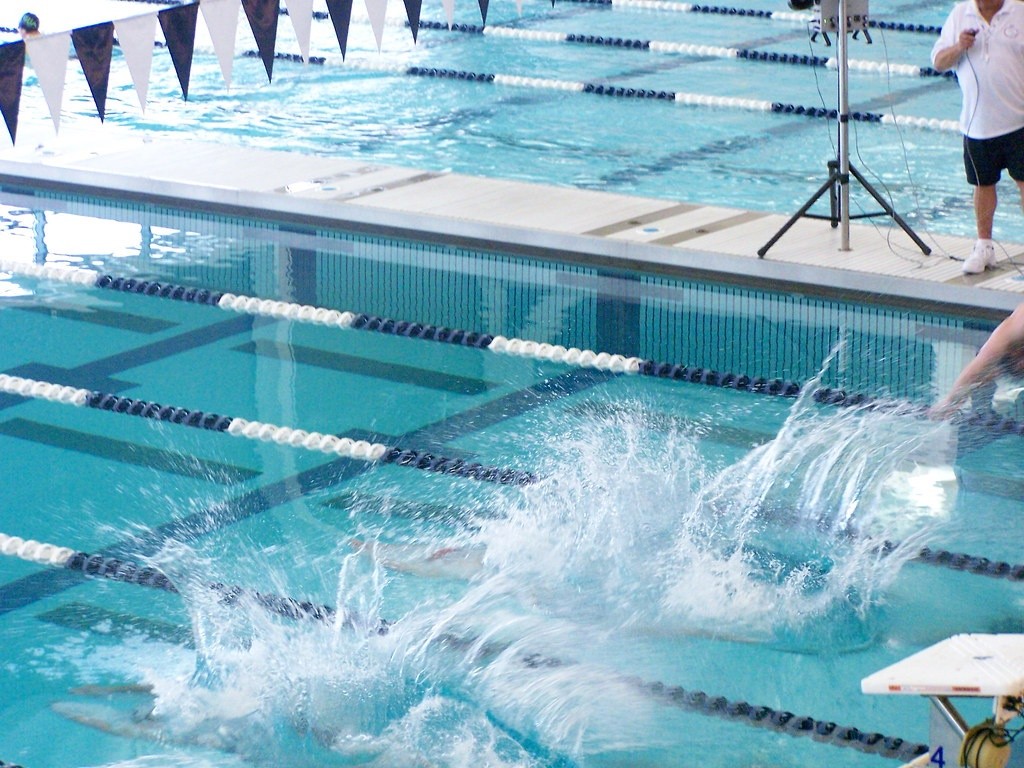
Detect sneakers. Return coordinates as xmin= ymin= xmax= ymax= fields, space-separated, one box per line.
xmin=961 ymin=243 xmax=996 ymax=273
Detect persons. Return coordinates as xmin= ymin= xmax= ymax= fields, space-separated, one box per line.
xmin=926 ymin=294 xmax=1024 ymax=424
xmin=929 ymin=0 xmax=1024 ymax=274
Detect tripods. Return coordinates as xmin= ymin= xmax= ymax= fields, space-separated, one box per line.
xmin=756 ymin=36 xmax=932 ymax=258
